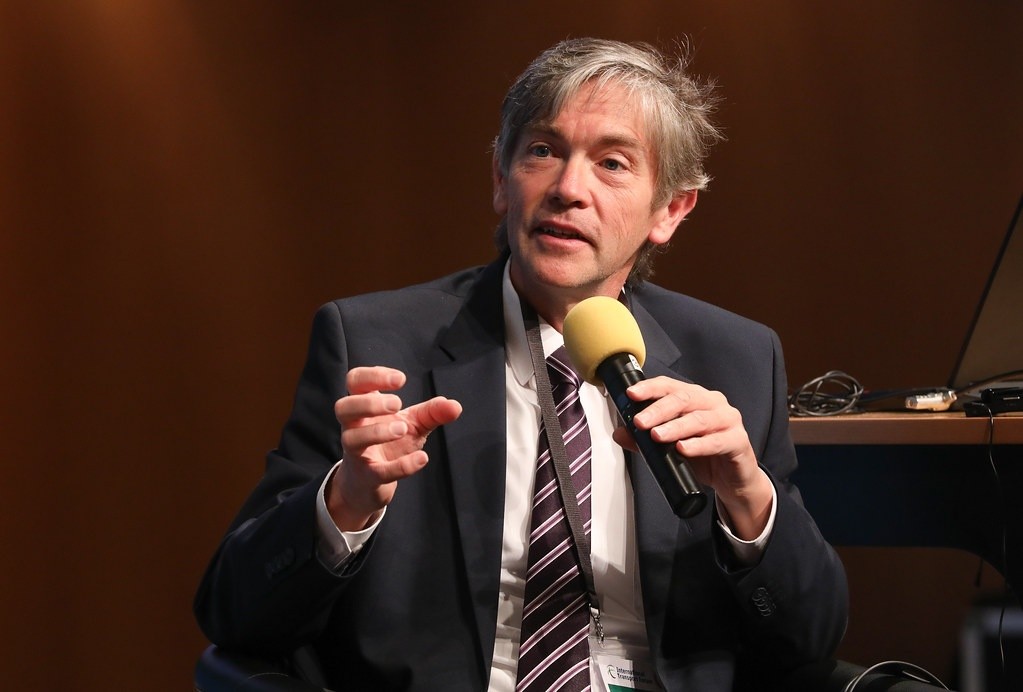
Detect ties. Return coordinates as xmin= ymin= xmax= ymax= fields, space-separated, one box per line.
xmin=515 ymin=344 xmax=592 ymax=692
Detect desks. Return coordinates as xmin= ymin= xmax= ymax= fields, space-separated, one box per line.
xmin=789 ymin=410 xmax=1023 ymax=446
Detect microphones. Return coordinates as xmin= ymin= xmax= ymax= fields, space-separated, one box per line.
xmin=562 ymin=296 xmax=708 ymax=519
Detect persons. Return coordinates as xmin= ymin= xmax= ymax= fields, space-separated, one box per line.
xmin=192 ymin=38 xmax=848 ymax=692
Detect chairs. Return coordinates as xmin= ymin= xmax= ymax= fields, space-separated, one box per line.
xmin=191 ymin=640 xmax=952 ymax=692
xmin=830 ymin=547 xmax=1010 ymax=682
